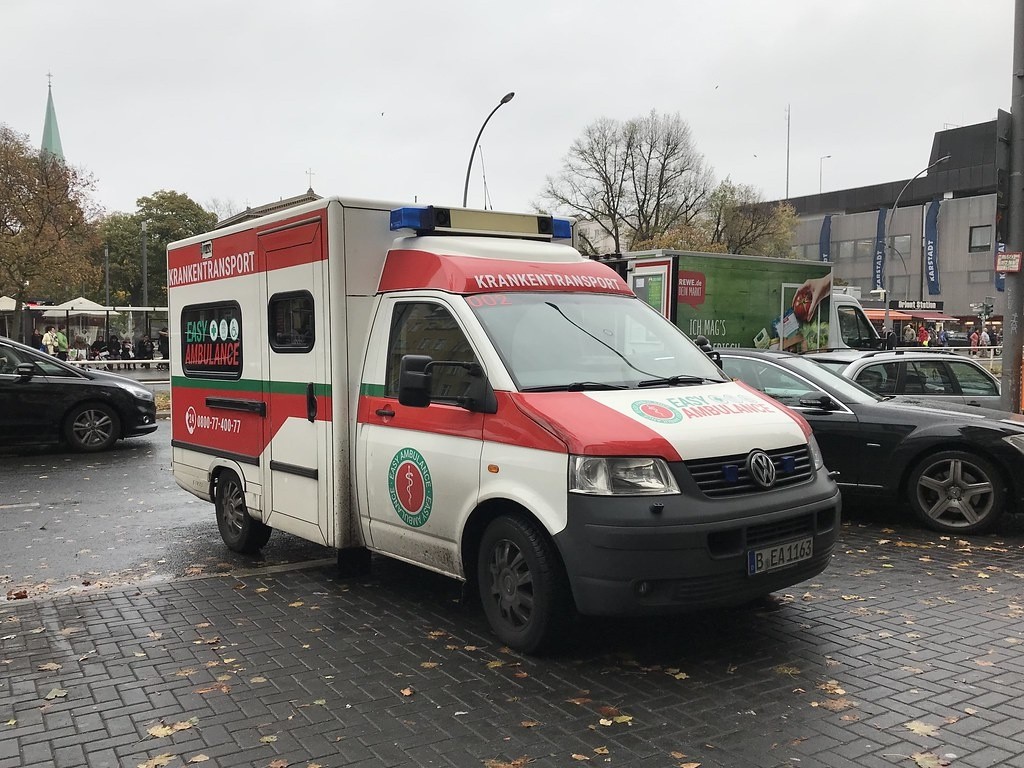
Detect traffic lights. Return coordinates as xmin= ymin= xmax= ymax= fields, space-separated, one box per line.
xmin=985 ymin=303 xmax=994 ymax=314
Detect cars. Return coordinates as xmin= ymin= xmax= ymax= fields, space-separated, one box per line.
xmin=779 ymin=348 xmax=1002 ymax=409
xmin=1 ymin=331 xmax=158 ymax=452
xmin=703 ymin=346 xmax=1024 ymax=536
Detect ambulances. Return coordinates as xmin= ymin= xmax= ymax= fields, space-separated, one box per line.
xmin=160 ymin=188 xmax=842 ymax=653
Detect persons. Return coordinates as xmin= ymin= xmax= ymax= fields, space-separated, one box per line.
xmin=792 ymin=273 xmax=831 ymax=322
xmin=32 ymin=325 xmax=169 ymax=370
xmin=904 ymin=324 xmax=1003 ymax=357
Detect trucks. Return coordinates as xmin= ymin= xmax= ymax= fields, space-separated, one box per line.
xmin=582 ymin=248 xmax=887 ymax=357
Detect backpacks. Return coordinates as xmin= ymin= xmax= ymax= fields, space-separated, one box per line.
xmin=940 ymin=332 xmax=947 ymax=342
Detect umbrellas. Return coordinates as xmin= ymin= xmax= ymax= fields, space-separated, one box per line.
xmin=0 ymin=296 xmax=28 ymax=338
xmin=41 ymin=297 xmax=122 ymax=335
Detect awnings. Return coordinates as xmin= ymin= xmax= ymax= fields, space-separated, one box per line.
xmin=864 ymin=309 xmax=912 ymax=321
xmin=899 ymin=311 xmax=961 ymax=323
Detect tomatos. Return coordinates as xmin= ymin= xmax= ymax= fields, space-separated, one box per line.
xmin=793 ymin=293 xmax=813 ymax=321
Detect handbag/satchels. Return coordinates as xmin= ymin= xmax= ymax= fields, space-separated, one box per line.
xmin=40 ymin=345 xmax=46 ymax=352
xmin=53 ymin=346 xmax=59 ymax=353
xmin=923 ymin=341 xmax=928 ymax=346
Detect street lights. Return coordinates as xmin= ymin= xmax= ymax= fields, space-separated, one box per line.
xmin=878 ymin=240 xmax=910 ymax=307
xmin=820 ymin=155 xmax=831 ymax=193
xmin=104 ymin=245 xmax=110 ymax=307
xmin=141 ymin=218 xmax=150 ymax=335
xmin=463 ymin=91 xmax=515 ymax=208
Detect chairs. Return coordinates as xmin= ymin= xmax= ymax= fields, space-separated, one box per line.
xmin=857 ymin=370 xmax=885 ymax=391
xmin=904 ymin=370 xmax=930 ymax=393
xmin=511 ymin=306 xmax=586 ymax=374
